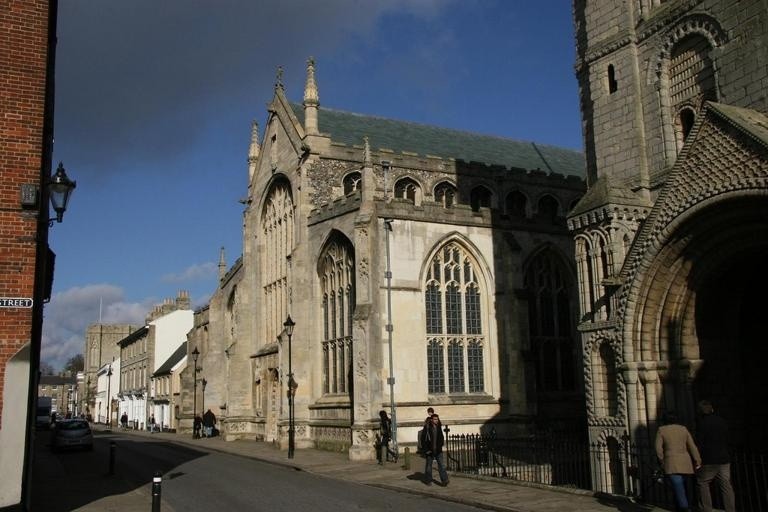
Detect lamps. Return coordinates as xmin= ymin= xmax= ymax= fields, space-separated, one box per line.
xmin=49 ymin=160 xmax=76 ymax=227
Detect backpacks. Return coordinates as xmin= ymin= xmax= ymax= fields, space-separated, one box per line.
xmin=418 ymin=429 xmax=428 ymax=450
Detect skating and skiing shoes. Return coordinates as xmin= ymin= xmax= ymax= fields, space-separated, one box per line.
xmin=443 ymin=480 xmax=449 ymax=487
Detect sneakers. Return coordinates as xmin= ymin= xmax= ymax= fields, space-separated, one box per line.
xmin=425 ymin=478 xmax=433 ymax=487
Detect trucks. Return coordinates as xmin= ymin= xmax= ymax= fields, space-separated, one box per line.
xmin=37 ymin=397 xmax=52 ymax=429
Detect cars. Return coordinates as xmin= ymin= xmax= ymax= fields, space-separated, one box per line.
xmin=54 ymin=419 xmax=93 ymax=453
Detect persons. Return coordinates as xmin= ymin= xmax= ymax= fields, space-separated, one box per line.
xmin=148 ymin=412 xmax=156 ymax=434
xmin=47 ymin=412 xmax=59 ymax=427
xmin=423 ymin=407 xmax=442 ymax=428
xmin=691 ymin=396 xmax=735 ymax=512
xmin=376 ymin=411 xmax=396 ymax=464
xmin=202 ymin=408 xmax=217 ymax=439
xmin=193 ymin=412 xmax=202 ymax=439
xmin=421 ymin=413 xmax=451 ymax=486
xmin=653 ymin=407 xmax=703 ymax=512
xmin=120 ymin=411 xmax=129 ymax=429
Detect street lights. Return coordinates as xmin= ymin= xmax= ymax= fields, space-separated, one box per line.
xmin=107 ymin=369 xmax=112 ymax=425
xmin=87 ymin=380 xmax=91 ymax=410
xmin=192 ymin=347 xmax=200 ymax=439
xmin=283 ymin=314 xmax=296 ymax=459
